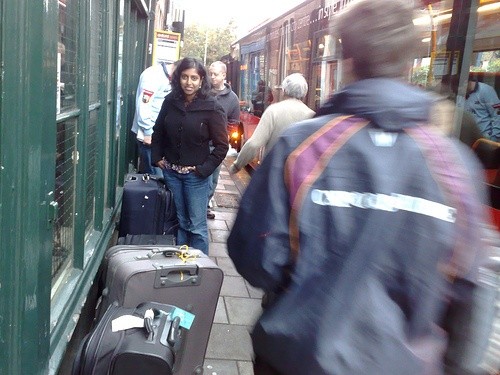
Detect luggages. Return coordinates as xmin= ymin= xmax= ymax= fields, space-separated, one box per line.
xmin=71 ymin=300 xmax=185 ymax=375
xmin=116 ymin=172 xmax=171 ymax=234
xmin=92 ymin=244 xmax=225 ymax=375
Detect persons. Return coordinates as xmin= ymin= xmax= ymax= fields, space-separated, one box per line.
xmin=251 ymin=81 xmax=273 ymax=117
xmin=229 ymin=73 xmax=317 ymax=174
xmin=462 ymin=76 xmax=500 ymax=142
xmin=203 ymin=61 xmax=241 ymax=220
xmin=226 ymin=0 xmax=485 ymax=375
xmin=131 ymin=59 xmax=183 ymax=174
xmin=151 ymin=56 xmax=228 ymax=255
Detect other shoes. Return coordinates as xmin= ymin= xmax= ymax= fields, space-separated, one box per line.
xmin=207 ymin=209 xmax=215 ymax=218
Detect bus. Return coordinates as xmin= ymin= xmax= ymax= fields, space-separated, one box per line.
xmin=219 ymin=0 xmax=500 ymax=246
